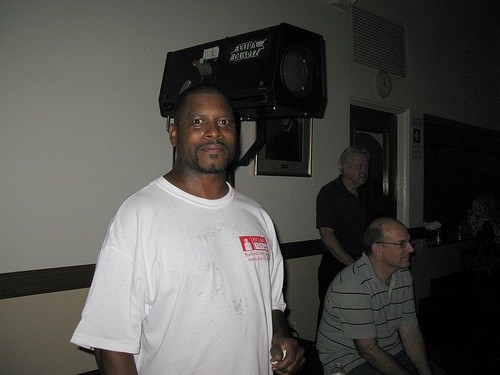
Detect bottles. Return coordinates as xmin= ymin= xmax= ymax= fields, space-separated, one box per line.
xmin=331 ymin=362 xmax=347 ymax=375
xmin=434 ymin=228 xmax=442 ymax=245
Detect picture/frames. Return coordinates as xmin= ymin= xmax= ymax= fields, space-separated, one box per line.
xmin=254 ymin=118 xmax=312 ymax=176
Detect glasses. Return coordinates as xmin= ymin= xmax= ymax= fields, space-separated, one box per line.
xmin=375 ymin=239 xmax=414 ymax=248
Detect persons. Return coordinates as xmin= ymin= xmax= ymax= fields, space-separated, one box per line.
xmin=314 ymin=214 xmax=435 ymax=375
xmin=315 ymin=144 xmax=386 ymax=338
xmin=70 ymin=84 xmax=312 ymax=375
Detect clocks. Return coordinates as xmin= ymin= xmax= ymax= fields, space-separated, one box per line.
xmin=375 ymin=71 xmax=391 ymax=97
